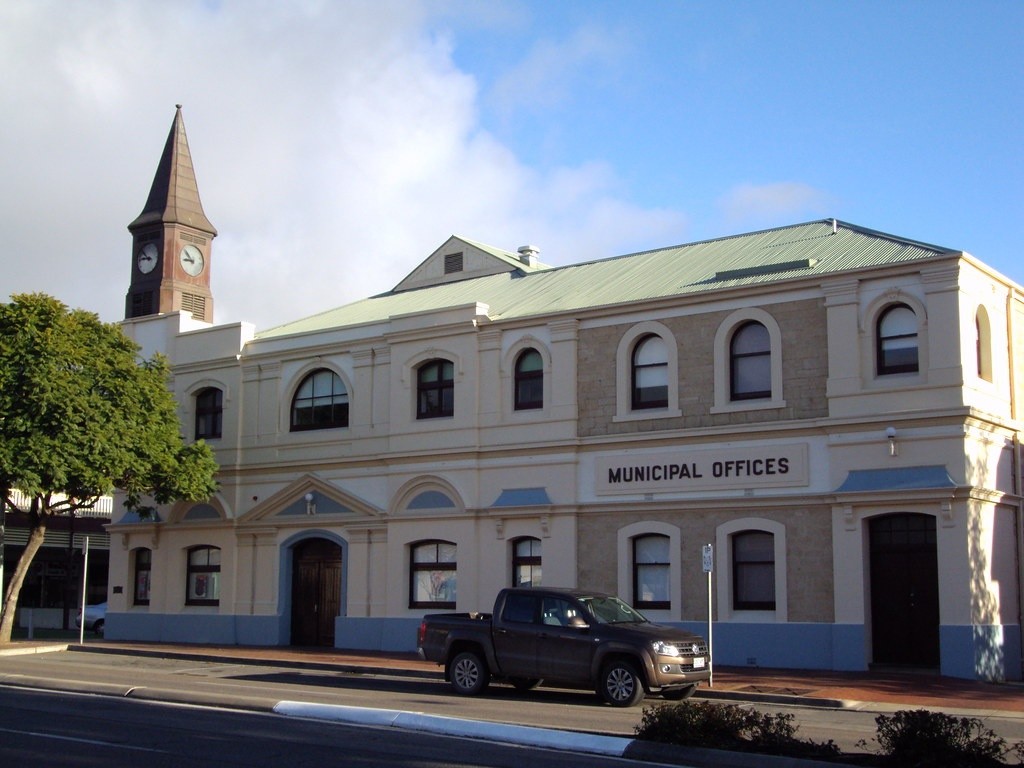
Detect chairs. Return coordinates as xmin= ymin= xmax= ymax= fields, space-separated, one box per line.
xmin=541 ymin=605 xmax=562 ymax=626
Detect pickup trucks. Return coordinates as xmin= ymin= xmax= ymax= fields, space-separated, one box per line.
xmin=415 ymin=585 xmax=712 ymax=708
